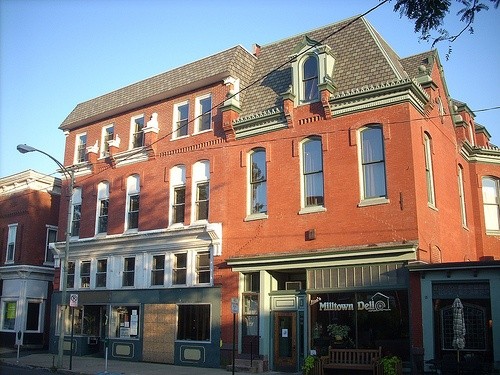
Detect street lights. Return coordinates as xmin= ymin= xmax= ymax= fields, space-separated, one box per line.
xmin=17 ymin=143 xmax=76 ymax=371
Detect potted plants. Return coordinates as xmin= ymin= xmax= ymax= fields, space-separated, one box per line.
xmin=320 ymin=346 xmax=382 ymax=375
xmin=302 ymin=354 xmax=320 ymax=375
xmin=376 ymin=356 xmax=403 ymax=375
xmin=327 ymin=324 xmax=351 ymax=340
